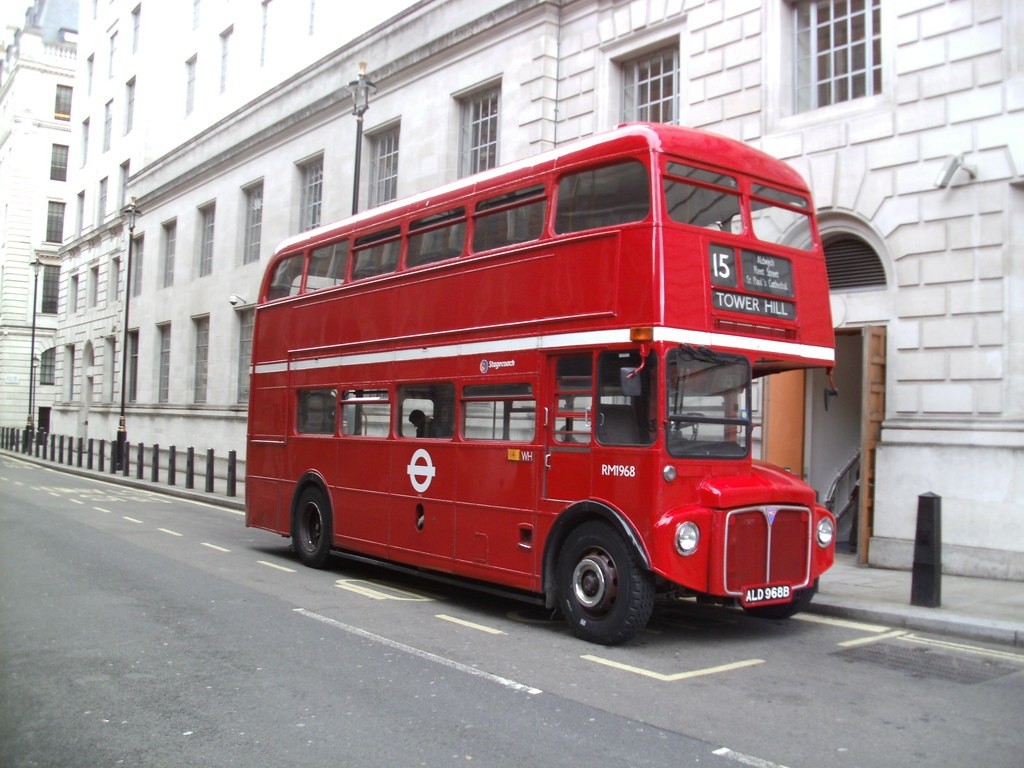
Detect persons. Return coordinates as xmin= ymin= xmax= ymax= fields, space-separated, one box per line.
xmin=409 ymin=410 xmax=441 ymax=438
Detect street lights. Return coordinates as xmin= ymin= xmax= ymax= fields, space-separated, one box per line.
xmin=114 ymin=197 xmax=142 ymax=470
xmin=343 ymin=61 xmax=378 ymax=215
xmin=23 ymin=254 xmax=43 ymax=439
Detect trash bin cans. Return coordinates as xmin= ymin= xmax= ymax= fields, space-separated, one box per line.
xmin=36 ymin=432 xmax=46 ymax=445
xmin=112 ymin=441 xmax=122 ymax=470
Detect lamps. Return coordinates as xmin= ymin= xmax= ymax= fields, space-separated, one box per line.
xmin=229 ymin=294 xmax=246 ymax=306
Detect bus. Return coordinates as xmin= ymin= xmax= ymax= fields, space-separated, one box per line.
xmin=242 ymin=118 xmax=841 ymax=646
